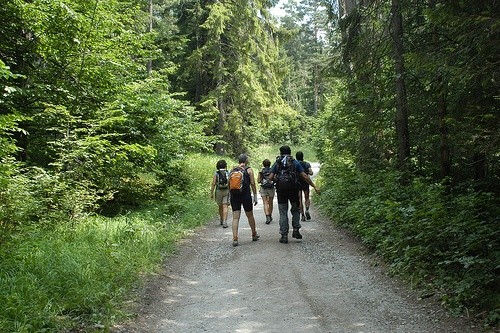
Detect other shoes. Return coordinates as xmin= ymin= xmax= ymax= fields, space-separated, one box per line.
xmin=221 ymin=219 xmax=223 ymax=225
xmin=233 ymin=240 xmax=238 ymax=246
xmin=252 ymin=235 xmax=259 ymax=241
xmin=266 ymin=214 xmax=272 ymax=224
xmin=301 ymin=216 xmax=305 ymax=220
xmin=305 ymin=211 xmax=311 ymax=220
xmin=292 ymin=230 xmax=302 ymax=239
xmin=223 ymin=224 xmax=228 ymax=228
xmin=279 ymin=234 xmax=288 ymax=243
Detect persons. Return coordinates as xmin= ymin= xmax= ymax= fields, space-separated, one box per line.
xmin=227 ymin=153 xmax=260 ymax=246
xmin=257 ymin=145 xmax=322 ymax=244
xmin=211 ymin=160 xmax=231 ymax=228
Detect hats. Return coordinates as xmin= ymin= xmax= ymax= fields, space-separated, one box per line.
xmin=263 ymin=159 xmax=270 ymax=166
xmin=218 ymin=160 xmax=227 ymax=168
xmin=238 ymin=154 xmax=248 ymax=163
xmin=279 ymin=146 xmax=290 ymax=154
xmin=296 ymin=152 xmax=303 ymax=159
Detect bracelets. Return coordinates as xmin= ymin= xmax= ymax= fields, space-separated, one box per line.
xmin=314 ymin=186 xmax=317 ymax=188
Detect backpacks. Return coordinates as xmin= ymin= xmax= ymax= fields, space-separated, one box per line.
xmin=216 ymin=170 xmax=230 ymax=191
xmin=261 ymin=166 xmax=274 ymax=189
xmin=274 ymin=154 xmax=298 ymax=196
xmin=297 ymin=161 xmax=309 ymax=189
xmin=228 ymin=166 xmax=251 ymax=195
